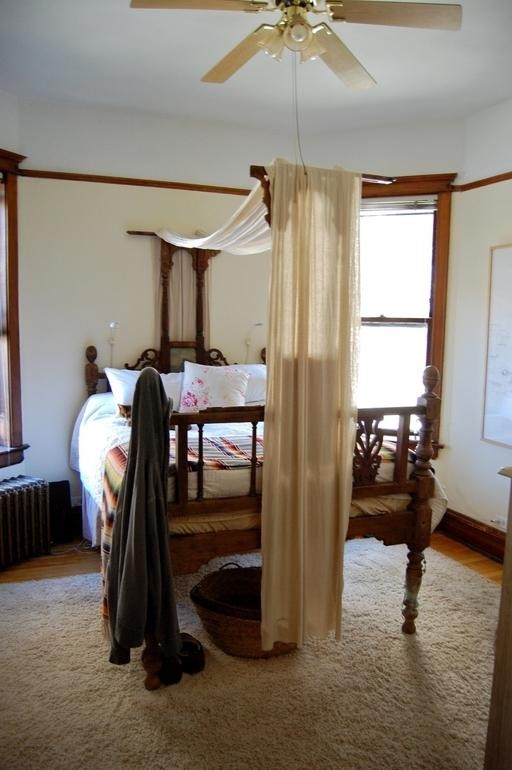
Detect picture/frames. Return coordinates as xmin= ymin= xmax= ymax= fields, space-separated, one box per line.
xmin=478 ymin=240 xmax=512 ymax=448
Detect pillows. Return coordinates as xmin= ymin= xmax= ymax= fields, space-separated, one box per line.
xmin=181 ymin=359 xmax=249 ymax=416
xmin=102 ymin=365 xmax=185 ymax=418
xmin=229 ymin=363 xmax=268 ymax=407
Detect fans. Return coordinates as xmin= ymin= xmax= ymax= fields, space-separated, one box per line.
xmin=124 ymin=0 xmax=466 ymax=91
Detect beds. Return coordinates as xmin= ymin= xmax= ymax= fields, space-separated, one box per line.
xmin=69 ymin=345 xmax=441 ymax=693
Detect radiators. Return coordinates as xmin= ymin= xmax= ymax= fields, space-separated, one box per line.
xmin=0 ymin=473 xmax=53 ymax=571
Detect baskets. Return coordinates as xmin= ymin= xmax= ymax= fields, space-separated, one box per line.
xmin=190 ymin=562 xmax=297 ymax=658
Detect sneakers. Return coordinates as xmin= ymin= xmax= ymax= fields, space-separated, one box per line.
xmin=142 ymin=633 xmax=205 ymax=684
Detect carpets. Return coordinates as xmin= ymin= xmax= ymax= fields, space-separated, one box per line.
xmin=1 ymin=532 xmax=500 ymax=769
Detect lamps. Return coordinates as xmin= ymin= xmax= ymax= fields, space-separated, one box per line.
xmin=244 ymin=0 xmax=343 ymax=64
